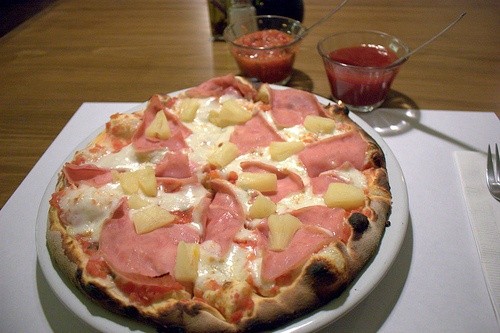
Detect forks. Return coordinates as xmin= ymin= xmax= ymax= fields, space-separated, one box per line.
xmin=486 ymin=145 xmax=500 ymax=200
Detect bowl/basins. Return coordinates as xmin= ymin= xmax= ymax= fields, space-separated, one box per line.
xmin=317 ymin=28 xmax=410 ymax=112
xmin=224 ymin=14 xmax=307 ymax=85
